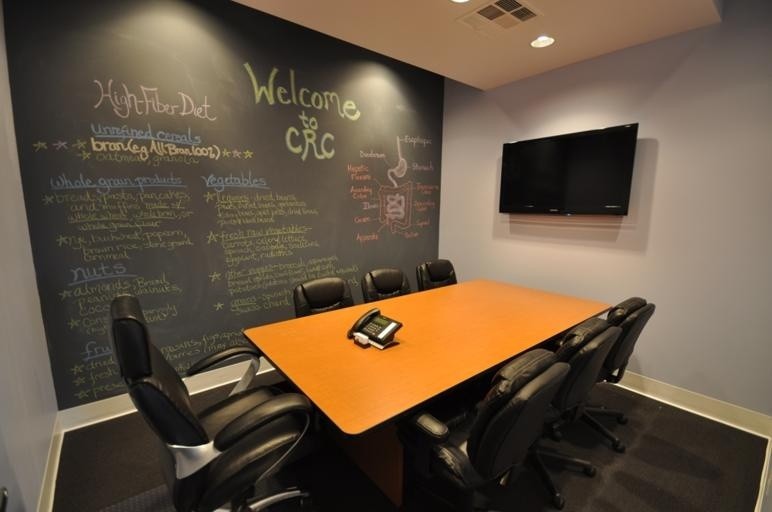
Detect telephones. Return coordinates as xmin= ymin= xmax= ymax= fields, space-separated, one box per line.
xmin=348 ymin=308 xmax=402 ymax=346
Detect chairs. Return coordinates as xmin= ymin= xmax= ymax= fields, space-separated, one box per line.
xmin=361 ymin=265 xmax=413 ymax=304
xmin=553 ymin=295 xmax=655 ymax=453
xmin=415 ymin=259 xmax=459 ymax=292
xmin=105 ymin=286 xmax=321 ymax=511
xmin=498 ymin=315 xmax=621 ymax=487
xmin=292 ymin=277 xmax=355 ymax=315
xmin=405 ymin=347 xmax=571 ymax=511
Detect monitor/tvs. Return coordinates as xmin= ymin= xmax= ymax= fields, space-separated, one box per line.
xmin=499 ymin=122 xmax=639 ymax=216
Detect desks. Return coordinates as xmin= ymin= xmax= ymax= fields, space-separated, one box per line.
xmin=240 ymin=280 xmax=616 ymax=509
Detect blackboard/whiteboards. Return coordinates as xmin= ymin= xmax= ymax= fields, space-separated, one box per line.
xmin=2 ymin=2 xmax=445 ymax=411
